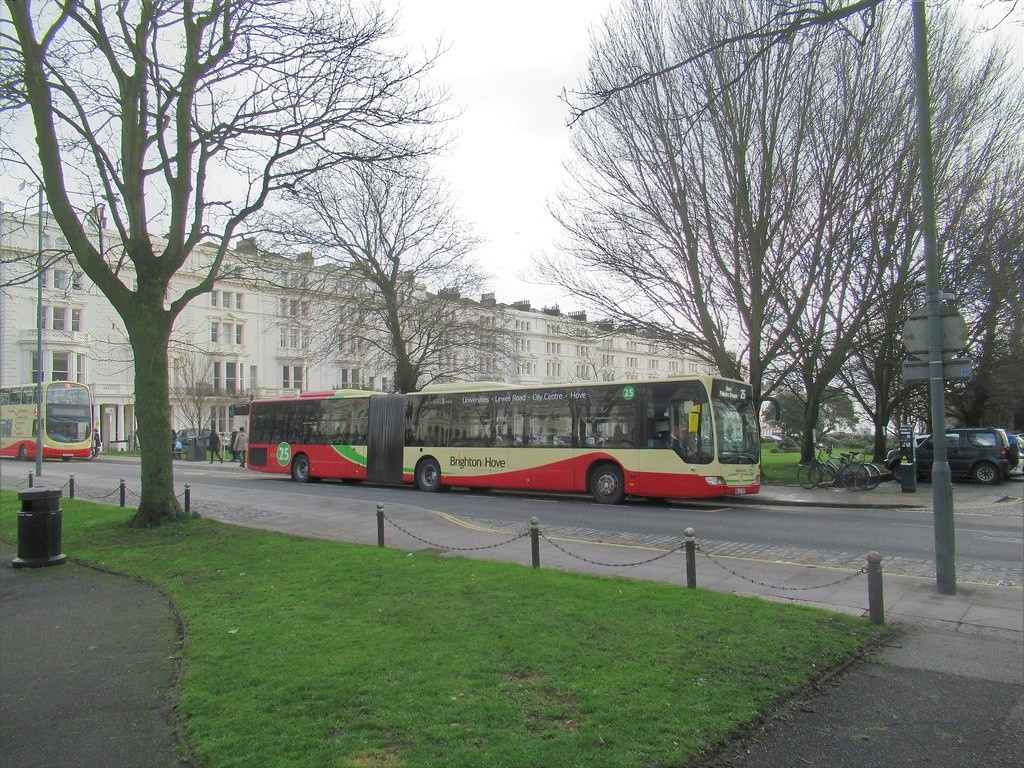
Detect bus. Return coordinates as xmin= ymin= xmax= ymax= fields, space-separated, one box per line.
xmin=246 ymin=375 xmax=763 ymax=506
xmin=0 ymin=380 xmax=96 ymax=462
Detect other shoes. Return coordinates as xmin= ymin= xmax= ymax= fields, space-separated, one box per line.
xmin=221 ymin=458 xmax=223 ymax=463
xmin=240 ymin=463 xmax=245 ymax=467
xmin=209 ymin=461 xmax=213 ymax=464
xmin=230 ymin=459 xmax=236 ymax=462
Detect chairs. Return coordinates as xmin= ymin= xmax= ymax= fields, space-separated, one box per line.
xmin=492 ymin=432 xmax=608 ymax=447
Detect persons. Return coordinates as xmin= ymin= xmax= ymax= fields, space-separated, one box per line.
xmin=229 ymin=427 xmax=242 ymax=462
xmin=94 ymin=429 xmax=101 ymax=458
xmin=171 ymin=430 xmax=177 ymax=451
xmin=233 ymin=426 xmax=249 ymax=467
xmin=208 ymin=429 xmax=223 ymax=464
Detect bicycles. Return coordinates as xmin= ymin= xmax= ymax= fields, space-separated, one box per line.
xmin=798 ymin=444 xmax=881 ymax=492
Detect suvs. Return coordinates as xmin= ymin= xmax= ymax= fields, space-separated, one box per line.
xmin=884 ymin=426 xmax=1019 ymax=485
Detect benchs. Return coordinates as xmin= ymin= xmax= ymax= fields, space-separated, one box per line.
xmin=172 ymin=450 xmax=185 ymax=459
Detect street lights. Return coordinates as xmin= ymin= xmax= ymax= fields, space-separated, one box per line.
xmin=35 ymin=185 xmax=122 ymax=476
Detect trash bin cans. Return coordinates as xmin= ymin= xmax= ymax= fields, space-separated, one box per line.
xmin=12 ymin=484 xmax=68 ymax=568
xmin=188 ymin=436 xmax=206 ymax=461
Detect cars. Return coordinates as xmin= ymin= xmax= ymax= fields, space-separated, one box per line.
xmin=494 ymin=433 xmax=608 ymax=449
xmin=1008 ymin=433 xmax=1024 ymax=473
xmin=176 ymin=428 xmax=233 ymax=452
xmin=914 ymin=432 xmax=993 ymax=449
xmin=695 ymin=435 xmax=783 ymax=445
